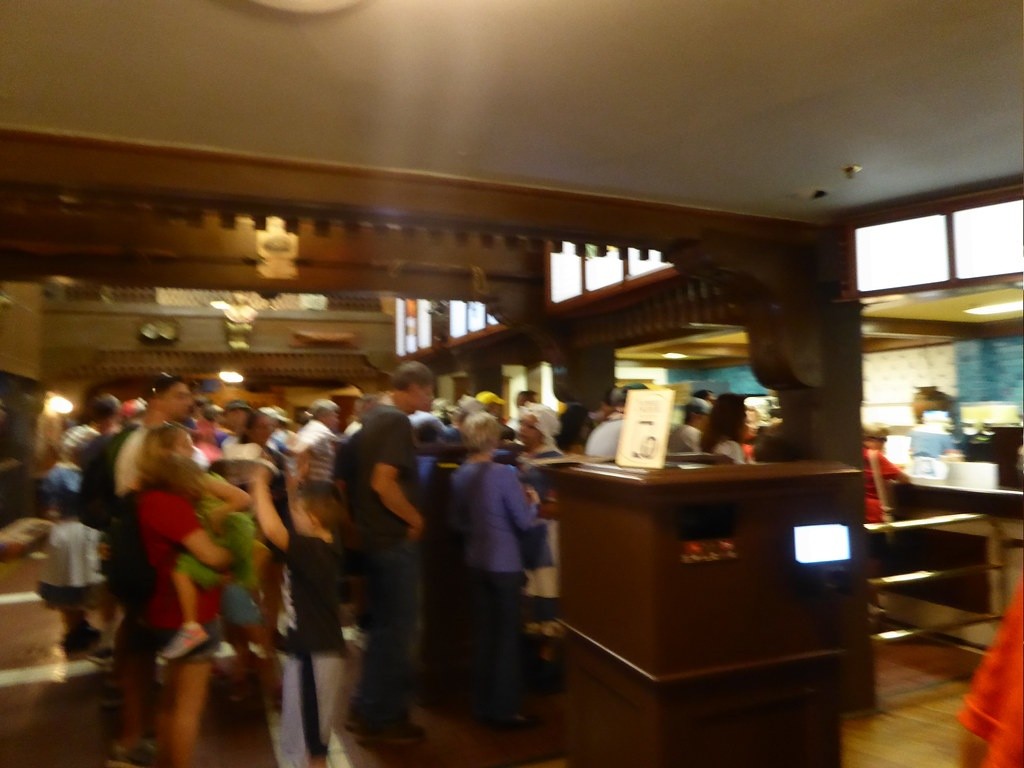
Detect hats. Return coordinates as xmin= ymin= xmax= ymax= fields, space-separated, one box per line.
xmin=620 ymin=382 xmax=651 ymax=398
xmin=475 ymin=390 xmax=507 ymax=406
xmin=685 ymin=397 xmax=713 ymax=416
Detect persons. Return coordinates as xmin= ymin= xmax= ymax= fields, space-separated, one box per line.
xmin=0 ymin=360 xmax=966 ymax=767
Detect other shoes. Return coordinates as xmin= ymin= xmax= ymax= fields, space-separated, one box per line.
xmin=60 ymin=621 xmax=102 ymax=653
xmin=104 ymin=738 xmax=158 ymax=768
xmin=480 ymin=706 xmax=546 ymax=732
xmin=343 ymin=720 xmax=425 ymax=746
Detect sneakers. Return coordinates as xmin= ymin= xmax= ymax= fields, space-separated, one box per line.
xmin=160 ymin=625 xmax=209 ymax=661
